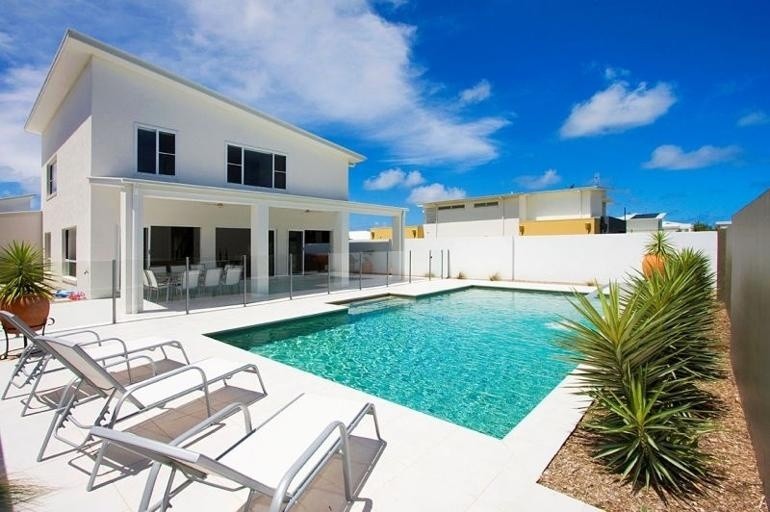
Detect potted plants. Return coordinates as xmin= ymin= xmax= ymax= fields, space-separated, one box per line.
xmin=0 ymin=237 xmax=62 ymax=334
xmin=641 ymin=230 xmax=678 ymax=278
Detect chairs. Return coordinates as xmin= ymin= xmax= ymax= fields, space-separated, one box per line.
xmin=142 ymin=264 xmax=243 ymax=305
xmin=86 ymin=392 xmax=381 ymax=512
xmin=32 ymin=355 xmax=268 ymax=492
xmin=2 ymin=330 xmax=186 ymax=416
